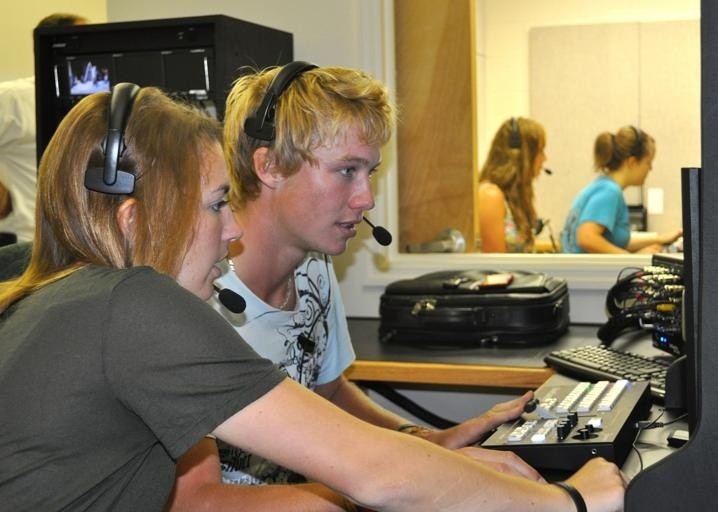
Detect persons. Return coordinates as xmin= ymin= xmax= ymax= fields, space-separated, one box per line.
xmin=558 ymin=125 xmax=683 ymax=255
xmin=477 ymin=116 xmax=562 ymax=256
xmin=0 ymin=83 xmax=631 ymax=512
xmin=165 ymin=60 xmax=549 ymax=512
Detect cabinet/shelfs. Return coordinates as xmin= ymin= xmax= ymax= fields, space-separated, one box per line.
xmin=33 ymin=13 xmax=293 ymax=203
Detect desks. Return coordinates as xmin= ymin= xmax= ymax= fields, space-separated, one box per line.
xmin=343 ymin=315 xmax=689 ymax=484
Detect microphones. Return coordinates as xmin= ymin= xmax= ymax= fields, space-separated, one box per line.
xmin=541 ymin=166 xmax=551 ymax=174
xmin=212 ymin=284 xmax=246 ymax=313
xmin=362 ymin=216 xmax=392 ymax=246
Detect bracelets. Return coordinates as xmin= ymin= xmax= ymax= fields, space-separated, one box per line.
xmin=397 ymin=422 xmax=420 ymax=432
xmin=553 ymin=479 xmax=591 ymax=512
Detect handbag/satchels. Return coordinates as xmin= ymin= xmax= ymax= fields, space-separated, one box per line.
xmin=378 ymin=267 xmax=570 ymax=347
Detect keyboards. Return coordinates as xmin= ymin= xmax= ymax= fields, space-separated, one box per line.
xmin=544 ymin=345 xmax=671 ymax=403
